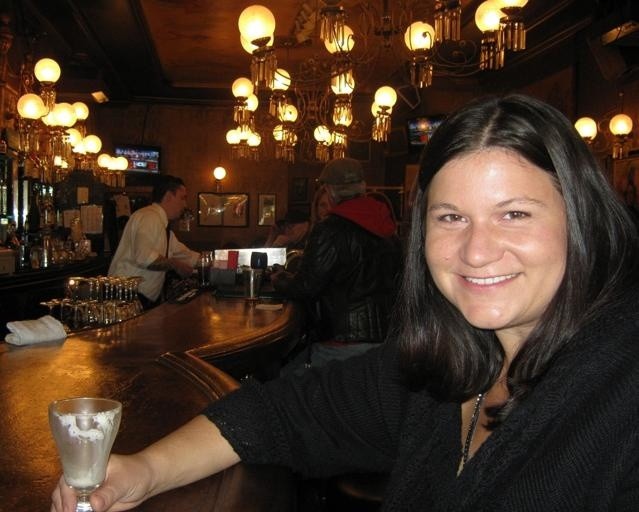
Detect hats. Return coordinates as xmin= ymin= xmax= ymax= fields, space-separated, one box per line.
xmin=315 ymin=157 xmax=365 ymax=186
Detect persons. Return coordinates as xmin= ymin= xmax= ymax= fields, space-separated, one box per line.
xmin=269 ymin=157 xmax=407 ymax=375
xmin=50 ymin=94 xmax=638 ymax=512
xmin=105 ymin=176 xmax=229 ymax=309
xmin=310 ymin=185 xmax=333 ymax=221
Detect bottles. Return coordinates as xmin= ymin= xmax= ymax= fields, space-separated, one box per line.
xmin=39 ymin=236 xmax=49 ymax=268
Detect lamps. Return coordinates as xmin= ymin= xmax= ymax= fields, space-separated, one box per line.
xmin=608 ymin=114 xmax=633 ymax=161
xmin=214 ymin=166 xmax=226 ymax=183
xmin=6 ymin=58 xmax=128 ymax=182
xmin=574 ymin=117 xmax=597 ymax=145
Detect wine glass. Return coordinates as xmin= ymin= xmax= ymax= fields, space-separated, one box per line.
xmin=39 ymin=272 xmax=145 ymax=334
xmin=46 ymin=396 xmax=124 ymax=512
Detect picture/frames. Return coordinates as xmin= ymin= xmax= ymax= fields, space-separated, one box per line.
xmin=197 ymin=191 xmax=249 ymax=228
xmin=258 ymin=192 xmax=276 ymax=227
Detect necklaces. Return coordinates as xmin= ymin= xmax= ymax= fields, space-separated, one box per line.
xmin=463 ymin=392 xmax=484 ymax=464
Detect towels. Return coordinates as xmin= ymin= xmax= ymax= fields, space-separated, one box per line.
xmin=5 ymin=315 xmax=66 ymax=347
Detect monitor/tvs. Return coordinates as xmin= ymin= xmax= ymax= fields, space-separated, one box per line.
xmin=113 ymin=143 xmax=160 ymax=176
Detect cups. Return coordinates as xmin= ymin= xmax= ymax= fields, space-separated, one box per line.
xmin=243 ymin=268 xmax=265 ymax=301
xmin=227 ymin=251 xmax=239 ymax=272
xmin=74 ymin=154 xmax=127 ymax=188
xmin=198 ymin=250 xmax=214 ymax=289
xmin=18 ymin=245 xmax=29 ymax=272
xmin=48 ymin=237 xmax=91 ymax=269
xmin=250 ymin=252 xmax=268 ymax=270
